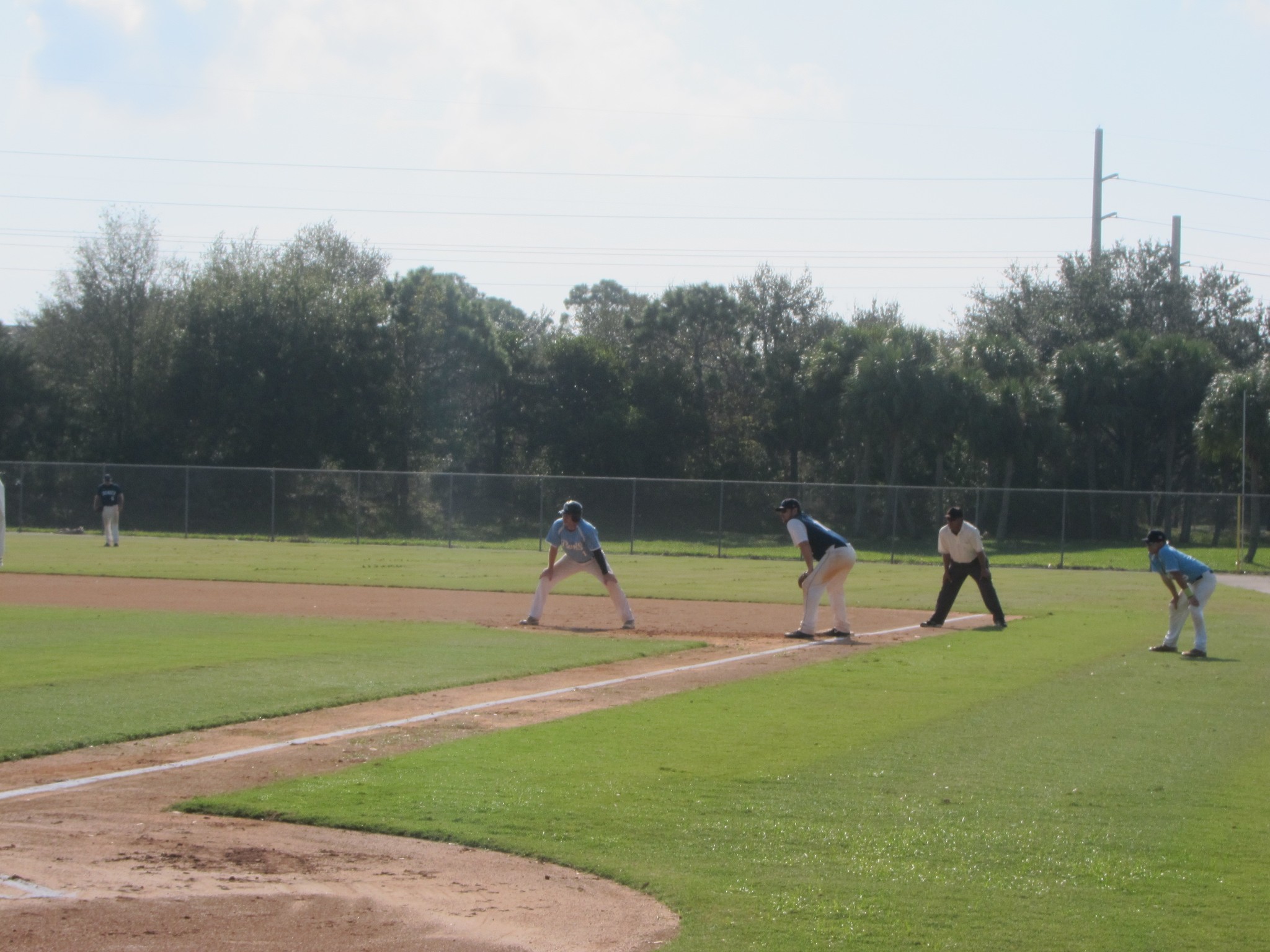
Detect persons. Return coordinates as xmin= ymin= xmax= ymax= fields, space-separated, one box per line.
xmin=93 ymin=474 xmax=123 ymax=547
xmin=519 ymin=500 xmax=635 ymax=629
xmin=776 ymin=498 xmax=857 ymax=638
xmin=920 ymin=506 xmax=1008 ymax=628
xmin=1141 ymin=530 xmax=1216 ymax=658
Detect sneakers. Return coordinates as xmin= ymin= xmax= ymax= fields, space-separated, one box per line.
xmin=995 ymin=622 xmax=1007 ymax=628
xmin=823 ymin=626 xmax=850 ymax=635
xmin=1182 ymin=649 xmax=1206 ymax=657
xmin=784 ymin=629 xmax=814 ymax=638
xmin=920 ymin=619 xmax=942 ymax=628
xmin=622 ymin=620 xmax=635 ymax=629
xmin=1149 ymin=645 xmax=1178 ymax=652
xmin=519 ymin=617 xmax=539 ymax=625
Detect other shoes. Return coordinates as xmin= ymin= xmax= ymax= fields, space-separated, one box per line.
xmin=104 ymin=543 xmax=111 ymax=546
xmin=114 ymin=543 xmax=118 ymax=546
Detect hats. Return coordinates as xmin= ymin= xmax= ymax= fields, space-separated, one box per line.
xmin=778 ymin=498 xmax=801 ymax=512
xmin=105 ymin=474 xmax=112 ymax=479
xmin=558 ymin=501 xmax=582 ymax=515
xmin=1142 ymin=530 xmax=1164 ymax=542
xmin=949 ymin=507 xmax=968 ymax=520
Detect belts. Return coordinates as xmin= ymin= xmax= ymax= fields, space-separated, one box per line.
xmin=1190 ymin=569 xmax=1213 ymax=584
